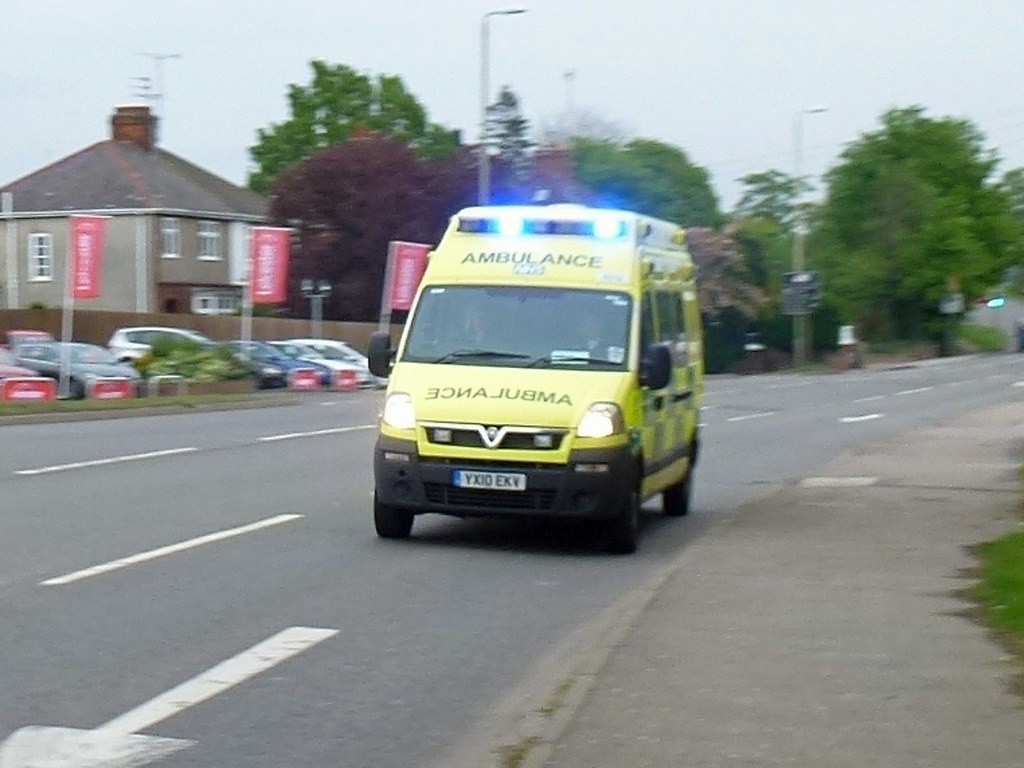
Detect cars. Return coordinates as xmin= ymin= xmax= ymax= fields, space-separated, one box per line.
xmin=0 ymin=325 xmax=394 ymax=400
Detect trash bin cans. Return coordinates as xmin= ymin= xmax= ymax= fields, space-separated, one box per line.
xmin=743 ymin=345 xmax=769 ymax=372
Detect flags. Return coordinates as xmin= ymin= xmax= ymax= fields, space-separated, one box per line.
xmin=392 ymin=245 xmax=427 ymax=310
xmin=252 ymin=230 xmax=289 ymax=302
xmin=70 ymin=217 xmax=105 ymax=298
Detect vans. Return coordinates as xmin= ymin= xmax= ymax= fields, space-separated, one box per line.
xmin=366 ymin=203 xmax=710 ymax=552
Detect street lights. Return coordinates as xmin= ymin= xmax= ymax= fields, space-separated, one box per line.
xmin=480 ymin=5 xmax=529 ymax=203
xmin=792 ymin=105 xmax=832 ymax=369
